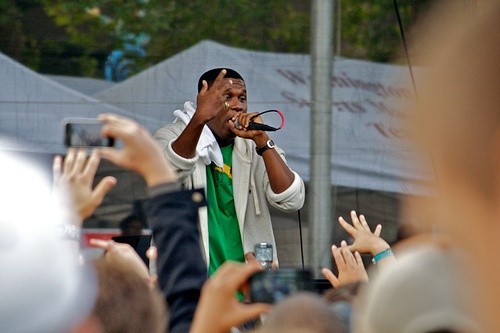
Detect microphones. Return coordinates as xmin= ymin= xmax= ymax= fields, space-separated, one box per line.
xmin=235 ymin=117 xmax=276 ymax=131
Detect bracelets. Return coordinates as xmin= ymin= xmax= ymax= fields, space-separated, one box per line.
xmin=371 ymin=248 xmax=393 ymax=264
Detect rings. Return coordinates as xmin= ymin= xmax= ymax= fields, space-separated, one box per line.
xmin=224 ymin=102 xmax=229 ymax=109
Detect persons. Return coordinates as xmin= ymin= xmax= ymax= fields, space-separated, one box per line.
xmin=0 ymin=113 xmax=455 ymax=333
xmin=154 ymin=68 xmax=305 ymax=333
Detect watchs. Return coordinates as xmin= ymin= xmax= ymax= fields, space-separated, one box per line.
xmin=255 ymin=139 xmax=275 ymax=156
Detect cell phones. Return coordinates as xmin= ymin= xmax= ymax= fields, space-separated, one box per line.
xmin=254 ymin=243 xmax=273 ymax=272
xmin=65 ymin=123 xmax=114 ymax=147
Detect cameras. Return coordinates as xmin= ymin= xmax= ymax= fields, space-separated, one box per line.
xmin=250 ymin=267 xmax=322 ymax=304
